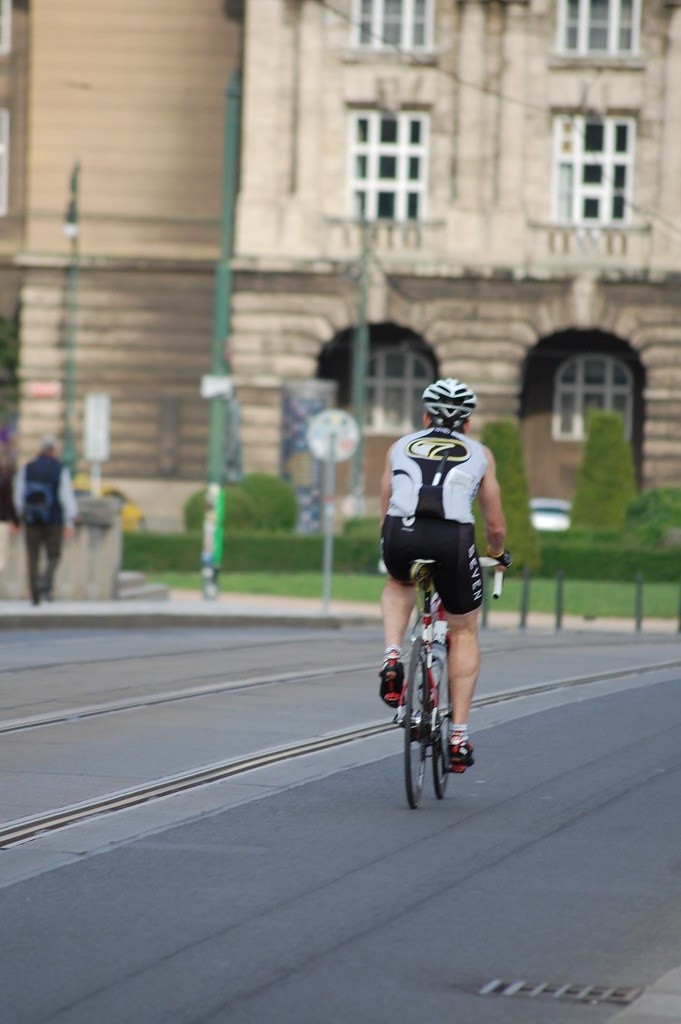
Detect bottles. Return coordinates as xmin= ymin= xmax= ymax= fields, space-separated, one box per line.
xmin=432 ymin=640 xmax=442 ymax=686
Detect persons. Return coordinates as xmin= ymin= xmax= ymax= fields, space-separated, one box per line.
xmin=0 ymin=435 xmax=79 ymax=605
xmin=376 ymin=377 xmax=511 ymax=766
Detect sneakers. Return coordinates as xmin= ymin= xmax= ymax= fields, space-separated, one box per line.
xmin=446 ymin=741 xmax=475 ymax=772
xmin=378 ymin=658 xmax=405 ymax=708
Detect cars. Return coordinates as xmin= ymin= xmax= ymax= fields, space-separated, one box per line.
xmin=528 ymin=496 xmax=573 ymax=533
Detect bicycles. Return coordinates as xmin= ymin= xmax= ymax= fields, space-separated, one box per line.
xmin=384 ymin=557 xmax=505 ymax=810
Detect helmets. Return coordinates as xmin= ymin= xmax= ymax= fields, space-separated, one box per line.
xmin=422 ymin=377 xmax=478 ymax=429
